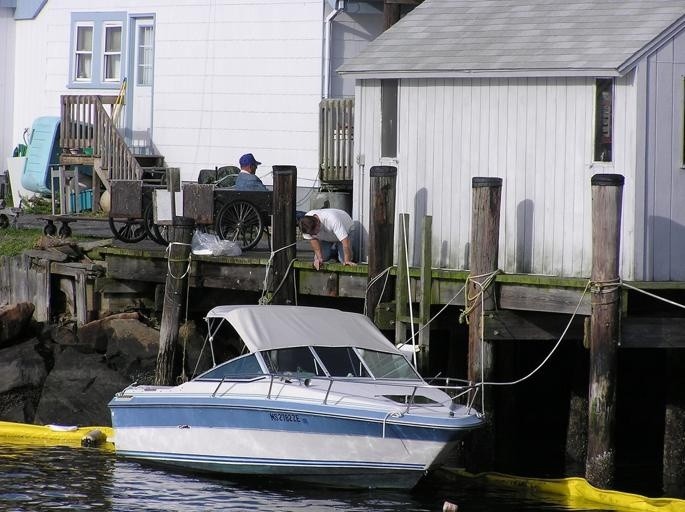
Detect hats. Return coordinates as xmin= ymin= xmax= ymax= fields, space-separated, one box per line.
xmin=237 ymin=152 xmax=262 ymax=168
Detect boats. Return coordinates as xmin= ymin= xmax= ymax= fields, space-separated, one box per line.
xmin=106 ymin=305 xmax=483 ymax=489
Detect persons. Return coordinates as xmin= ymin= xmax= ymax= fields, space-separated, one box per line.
xmin=235 ymin=153 xmax=331 ymax=227
xmin=298 ymin=207 xmax=358 ymax=271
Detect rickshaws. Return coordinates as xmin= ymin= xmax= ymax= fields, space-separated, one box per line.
xmin=108 ymin=180 xmax=273 ymax=251
